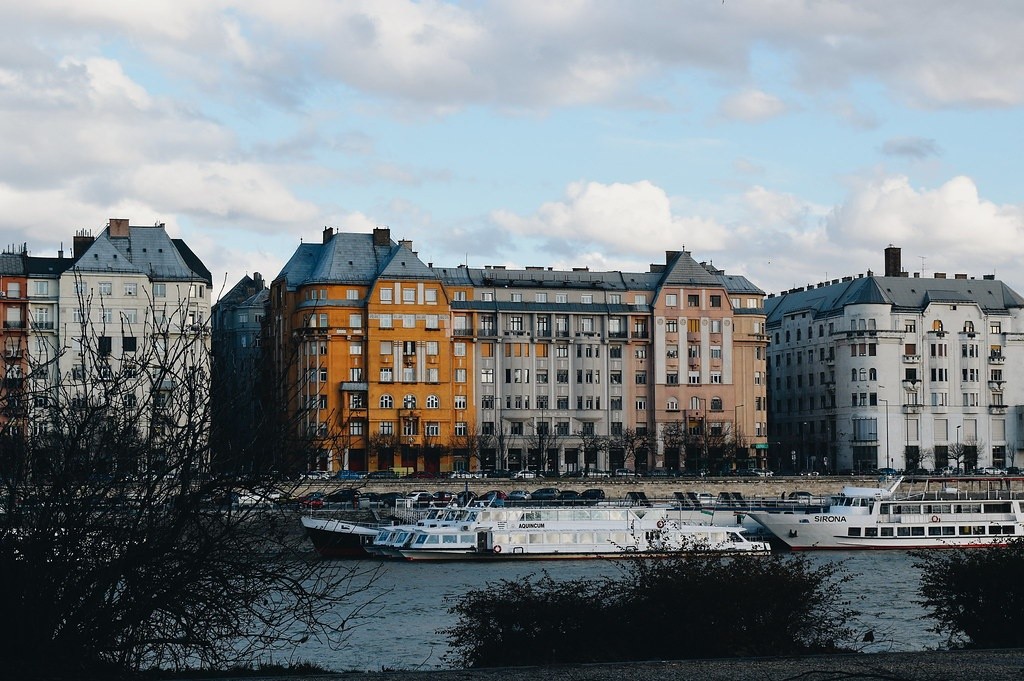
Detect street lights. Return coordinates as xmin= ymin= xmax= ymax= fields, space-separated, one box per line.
xmin=957 ymin=425 xmax=961 ymax=476
xmin=494 ymin=397 xmax=504 ymax=470
xmin=878 ymin=398 xmax=889 ymax=468
xmin=696 ymin=397 xmax=707 ymax=474
xmin=734 ymin=404 xmax=744 ymax=454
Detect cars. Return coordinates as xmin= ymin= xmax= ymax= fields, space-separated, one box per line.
xmin=854 ymin=466 xmax=1024 ymax=477
xmin=119 ymin=465 xmax=825 ymax=483
xmin=295 ymin=487 xmax=606 ymax=507
xmin=788 ymin=491 xmax=814 ymax=501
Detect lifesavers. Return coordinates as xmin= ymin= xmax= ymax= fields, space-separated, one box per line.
xmin=493 ymin=544 xmax=502 ymax=553
xmin=657 ymin=521 xmax=664 ymax=528
xmin=931 ymin=515 xmax=939 ymax=522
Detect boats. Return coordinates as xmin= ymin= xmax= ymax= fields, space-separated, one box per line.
xmin=363 ymin=493 xmax=774 ymax=558
xmin=744 ymin=473 xmax=1024 ymax=551
xmin=301 ymin=515 xmax=380 ymax=560
